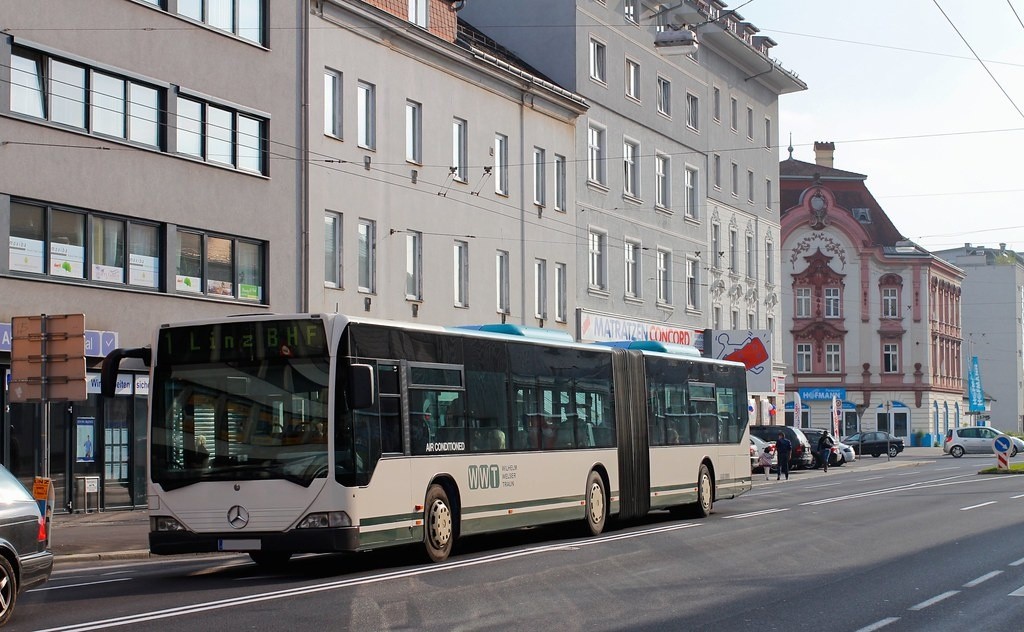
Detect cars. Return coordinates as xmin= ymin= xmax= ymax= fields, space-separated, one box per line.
xmin=943 ymin=426 xmax=1024 ymax=458
xmin=750 ymin=434 xmax=779 ymax=472
xmin=0 ymin=460 xmax=54 ymax=627
xmin=841 ymin=431 xmax=905 ymax=457
xmin=800 ymin=431 xmax=842 ymax=470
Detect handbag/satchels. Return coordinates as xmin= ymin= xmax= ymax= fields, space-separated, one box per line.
xmin=823 ymin=438 xmax=833 ymax=447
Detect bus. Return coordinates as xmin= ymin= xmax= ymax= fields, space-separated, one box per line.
xmin=100 ymin=312 xmax=753 ymax=569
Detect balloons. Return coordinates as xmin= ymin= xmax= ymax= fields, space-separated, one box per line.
xmin=768 ymin=403 xmax=777 ymax=416
xmin=750 ymin=408 xmax=754 ymax=412
xmin=749 ymin=405 xmax=752 ymax=411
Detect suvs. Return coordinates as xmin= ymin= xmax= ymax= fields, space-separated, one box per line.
xmin=799 ymin=427 xmax=856 ymax=467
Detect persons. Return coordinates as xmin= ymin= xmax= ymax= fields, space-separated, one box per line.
xmin=772 ymin=432 xmax=792 ymax=481
xmin=758 ymin=447 xmax=776 ymax=481
xmin=194 ymin=434 xmax=210 ymax=468
xmin=425 ymin=387 xmax=723 ymax=452
xmin=313 ymin=423 xmax=325 ymax=438
xmin=84 ymin=435 xmax=92 ymax=458
xmin=817 ymin=431 xmax=835 ymax=473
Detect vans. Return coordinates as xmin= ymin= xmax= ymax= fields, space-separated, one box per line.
xmin=749 ymin=425 xmax=813 ymax=471
